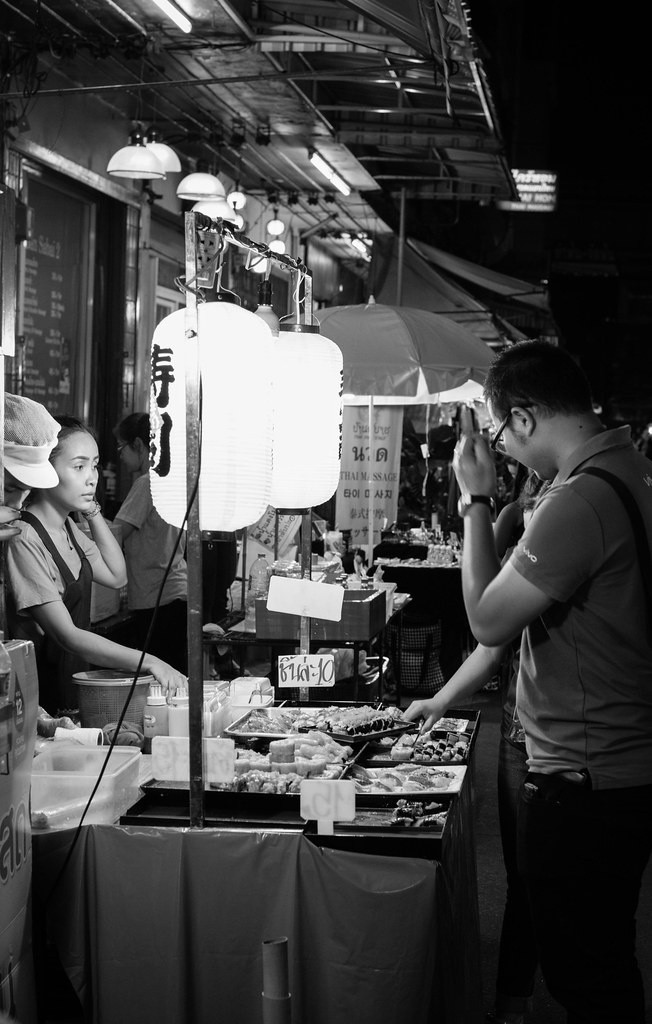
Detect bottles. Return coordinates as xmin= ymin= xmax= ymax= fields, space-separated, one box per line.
xmin=428 ymin=543 xmax=455 ymax=566
xmin=169 ymin=689 xmax=191 ymax=736
xmin=333 ymin=572 xmax=375 ymax=591
xmin=144 ymin=685 xmax=168 ymax=738
xmin=245 ymin=554 xmax=269 ymax=630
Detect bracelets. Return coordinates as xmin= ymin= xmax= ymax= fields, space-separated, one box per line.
xmin=81 ymin=501 xmax=101 ymax=521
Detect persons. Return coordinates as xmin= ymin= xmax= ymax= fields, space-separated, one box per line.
xmin=403 ymin=339 xmax=652 ymax=1024
xmin=0 ymin=392 xmax=191 ymax=741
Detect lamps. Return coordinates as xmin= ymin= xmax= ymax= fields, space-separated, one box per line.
xmin=106 ymin=47 xmax=245 ymax=234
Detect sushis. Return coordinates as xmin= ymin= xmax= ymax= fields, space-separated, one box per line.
xmin=210 ymin=705 xmax=471 ymax=826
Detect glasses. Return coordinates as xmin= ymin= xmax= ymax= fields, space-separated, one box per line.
xmin=491 ymin=401 xmax=534 ymax=458
xmin=114 ymin=439 xmax=133 ymax=454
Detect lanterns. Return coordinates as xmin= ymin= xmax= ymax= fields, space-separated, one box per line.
xmin=276 ymin=324 xmax=344 ymax=514
xmin=149 ymin=293 xmax=277 ymax=543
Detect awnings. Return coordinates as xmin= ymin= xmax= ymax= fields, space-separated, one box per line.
xmin=373 ymin=232 xmax=551 ymax=345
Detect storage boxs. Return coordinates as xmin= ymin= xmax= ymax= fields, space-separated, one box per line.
xmin=29 ymin=744 xmax=142 ymax=833
xmin=255 ymin=580 xmax=397 ymax=642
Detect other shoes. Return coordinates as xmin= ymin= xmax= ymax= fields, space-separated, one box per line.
xmin=486 ymin=1011 xmax=524 ymax=1024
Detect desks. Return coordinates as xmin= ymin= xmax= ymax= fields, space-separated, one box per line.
xmin=363 ymin=563 xmax=472 ymax=707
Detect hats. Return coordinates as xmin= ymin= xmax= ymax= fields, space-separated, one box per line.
xmin=3 ymin=392 xmax=61 ymax=488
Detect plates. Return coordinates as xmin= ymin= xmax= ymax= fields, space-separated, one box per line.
xmin=349 ymin=764 xmax=468 ymax=794
xmin=298 ymin=721 xmax=419 ymax=743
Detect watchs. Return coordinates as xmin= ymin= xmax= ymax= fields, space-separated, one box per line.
xmin=457 ymin=494 xmax=497 ymax=522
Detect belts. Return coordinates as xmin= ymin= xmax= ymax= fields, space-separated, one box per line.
xmin=500 ymin=710 xmax=527 ymax=755
xmin=554 ymin=771 xmax=592 ymax=786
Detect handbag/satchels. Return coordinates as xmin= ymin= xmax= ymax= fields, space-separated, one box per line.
xmin=384 ymin=617 xmax=446 ymax=696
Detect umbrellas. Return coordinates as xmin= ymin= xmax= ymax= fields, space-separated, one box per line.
xmin=279 ymin=293 xmax=501 ymax=566
